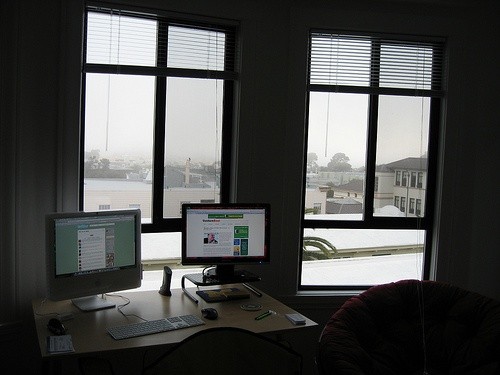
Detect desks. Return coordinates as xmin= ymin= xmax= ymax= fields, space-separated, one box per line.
xmin=30 ymin=283 xmax=318 ymax=375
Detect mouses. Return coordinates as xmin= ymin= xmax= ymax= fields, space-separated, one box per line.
xmin=201 ymin=307 xmax=218 ymax=320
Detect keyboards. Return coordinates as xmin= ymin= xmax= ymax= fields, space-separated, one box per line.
xmin=106 ymin=314 xmax=206 ymax=341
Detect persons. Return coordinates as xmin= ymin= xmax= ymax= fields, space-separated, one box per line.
xmin=204 ymin=231 xmax=218 ymax=244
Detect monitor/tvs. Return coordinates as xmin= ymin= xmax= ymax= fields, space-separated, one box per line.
xmin=180 ymin=203 xmax=271 ymax=282
xmin=44 ymin=209 xmax=142 ymax=312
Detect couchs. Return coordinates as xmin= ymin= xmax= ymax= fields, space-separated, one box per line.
xmin=316 ymin=279 xmax=500 ymax=375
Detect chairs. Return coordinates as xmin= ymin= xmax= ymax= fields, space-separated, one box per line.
xmin=144 ymin=326 xmax=304 ymax=375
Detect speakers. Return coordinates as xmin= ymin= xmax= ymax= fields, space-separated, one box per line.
xmin=158 ymin=265 xmax=172 ymax=296
